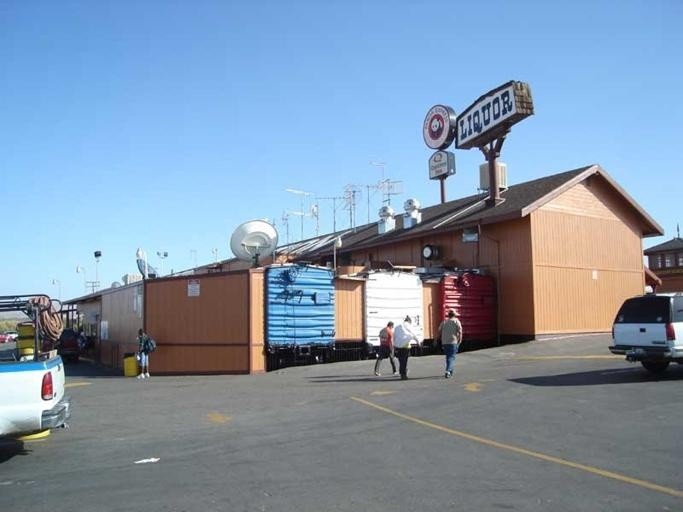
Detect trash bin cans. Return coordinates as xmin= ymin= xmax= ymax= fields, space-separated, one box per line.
xmin=123 ymin=352 xmax=139 ymax=377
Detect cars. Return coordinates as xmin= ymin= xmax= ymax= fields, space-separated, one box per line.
xmin=2 ymin=330 xmax=17 ymax=342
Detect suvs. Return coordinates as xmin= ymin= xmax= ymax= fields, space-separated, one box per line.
xmin=0 ymin=292 xmax=75 ymax=462
xmin=607 ymin=291 xmax=683 ymax=374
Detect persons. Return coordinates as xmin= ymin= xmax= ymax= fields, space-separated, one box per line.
xmin=136 ymin=329 xmax=150 ymax=379
xmin=375 ymin=317 xmax=420 ymax=380
xmin=438 ymin=310 xmax=462 ymax=378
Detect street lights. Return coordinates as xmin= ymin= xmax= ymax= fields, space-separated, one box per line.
xmin=76 ymin=265 xmax=87 ymax=297
xmin=52 ymin=278 xmax=61 ymax=301
xmin=93 ymin=249 xmax=103 ymax=293
xmin=157 ymin=250 xmax=168 ymax=275
xmin=371 ymin=160 xmax=389 ymax=210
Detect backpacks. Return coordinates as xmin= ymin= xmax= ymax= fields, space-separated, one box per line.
xmin=140 ymin=337 xmax=156 ymax=353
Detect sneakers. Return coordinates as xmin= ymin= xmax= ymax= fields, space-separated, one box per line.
xmin=375 ymin=371 xmax=380 ymax=376
xmin=401 ymin=375 xmax=408 ymax=379
xmin=393 ymin=371 xmax=399 ymax=376
xmin=445 ymin=371 xmax=454 ymax=378
xmin=137 ymin=372 xmax=150 ymax=380
